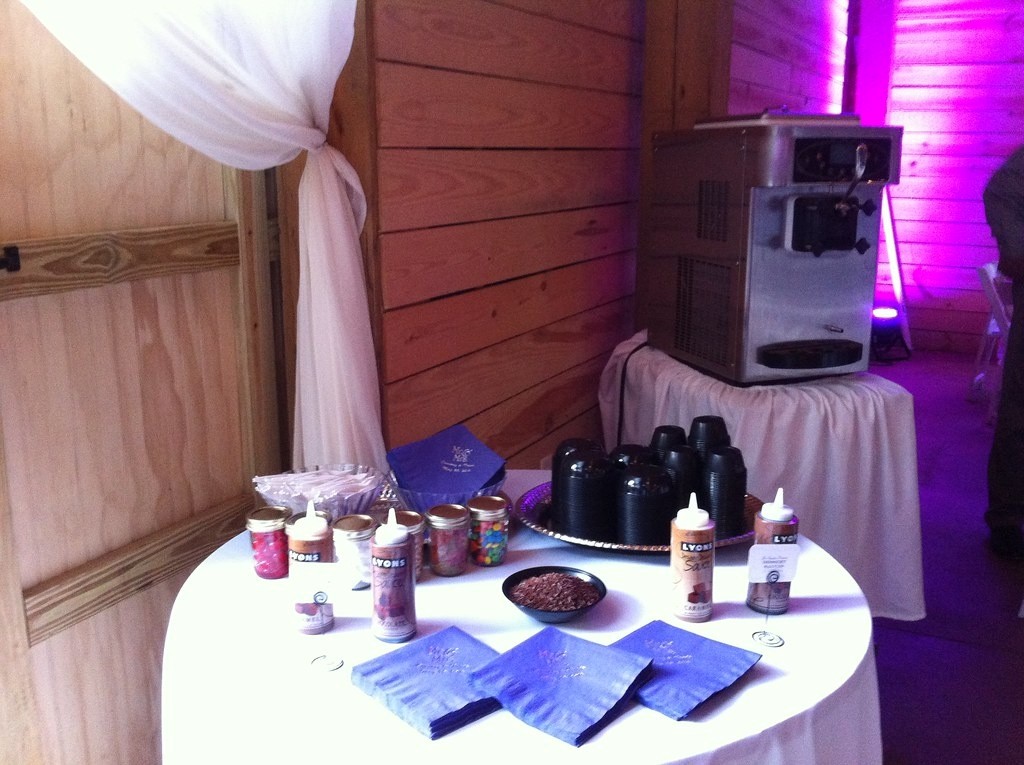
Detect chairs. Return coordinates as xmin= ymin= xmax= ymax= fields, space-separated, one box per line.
xmin=971 ymin=261 xmax=1019 ymax=411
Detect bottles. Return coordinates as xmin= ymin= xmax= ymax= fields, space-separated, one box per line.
xmin=745 ymin=488 xmax=799 ymax=615
xmin=671 ymin=493 xmax=715 ymax=622
xmin=371 ymin=508 xmax=416 ymax=643
xmin=288 ymin=499 xmax=335 ymax=635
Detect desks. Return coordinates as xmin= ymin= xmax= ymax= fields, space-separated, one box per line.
xmin=160 ymin=470 xmax=883 ymax=765
xmin=599 ymin=331 xmax=928 ymax=621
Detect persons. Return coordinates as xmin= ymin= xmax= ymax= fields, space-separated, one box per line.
xmin=984 ymin=147 xmax=1024 ymax=565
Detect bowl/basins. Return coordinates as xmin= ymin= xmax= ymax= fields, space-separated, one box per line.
xmin=552 ymin=414 xmax=748 ymax=545
xmin=258 ymin=465 xmax=386 ymax=519
xmin=387 ymin=469 xmax=506 ymax=517
xmin=502 ymin=566 xmax=606 ymax=623
